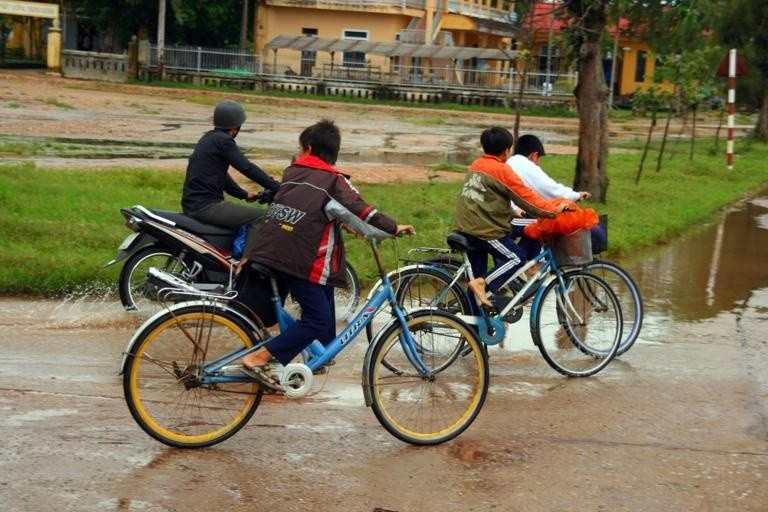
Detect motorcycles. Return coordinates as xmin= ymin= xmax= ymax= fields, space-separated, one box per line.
xmin=100 ymin=185 xmax=361 ymax=326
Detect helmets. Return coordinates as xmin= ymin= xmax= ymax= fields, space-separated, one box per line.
xmin=213 ymin=99 xmax=249 ymax=129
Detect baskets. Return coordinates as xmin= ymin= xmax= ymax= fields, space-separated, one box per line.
xmin=591 ymin=213 xmax=608 ymax=254
xmin=542 ymin=226 xmax=593 ymax=266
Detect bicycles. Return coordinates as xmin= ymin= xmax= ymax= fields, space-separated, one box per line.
xmin=366 ymin=204 xmax=623 ymax=378
xmin=393 ymin=192 xmax=645 ymax=358
xmin=116 ymin=225 xmax=491 ymax=446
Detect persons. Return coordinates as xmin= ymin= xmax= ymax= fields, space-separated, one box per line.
xmin=506 ymin=135 xmax=592 ymax=278
xmin=452 ymin=126 xmax=570 ymax=309
xmin=240 ymin=115 xmax=417 ymax=395
xmin=181 ymin=99 xmax=283 ymax=279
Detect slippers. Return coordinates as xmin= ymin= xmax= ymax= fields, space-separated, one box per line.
xmin=240 ymin=362 xmax=285 ymax=393
